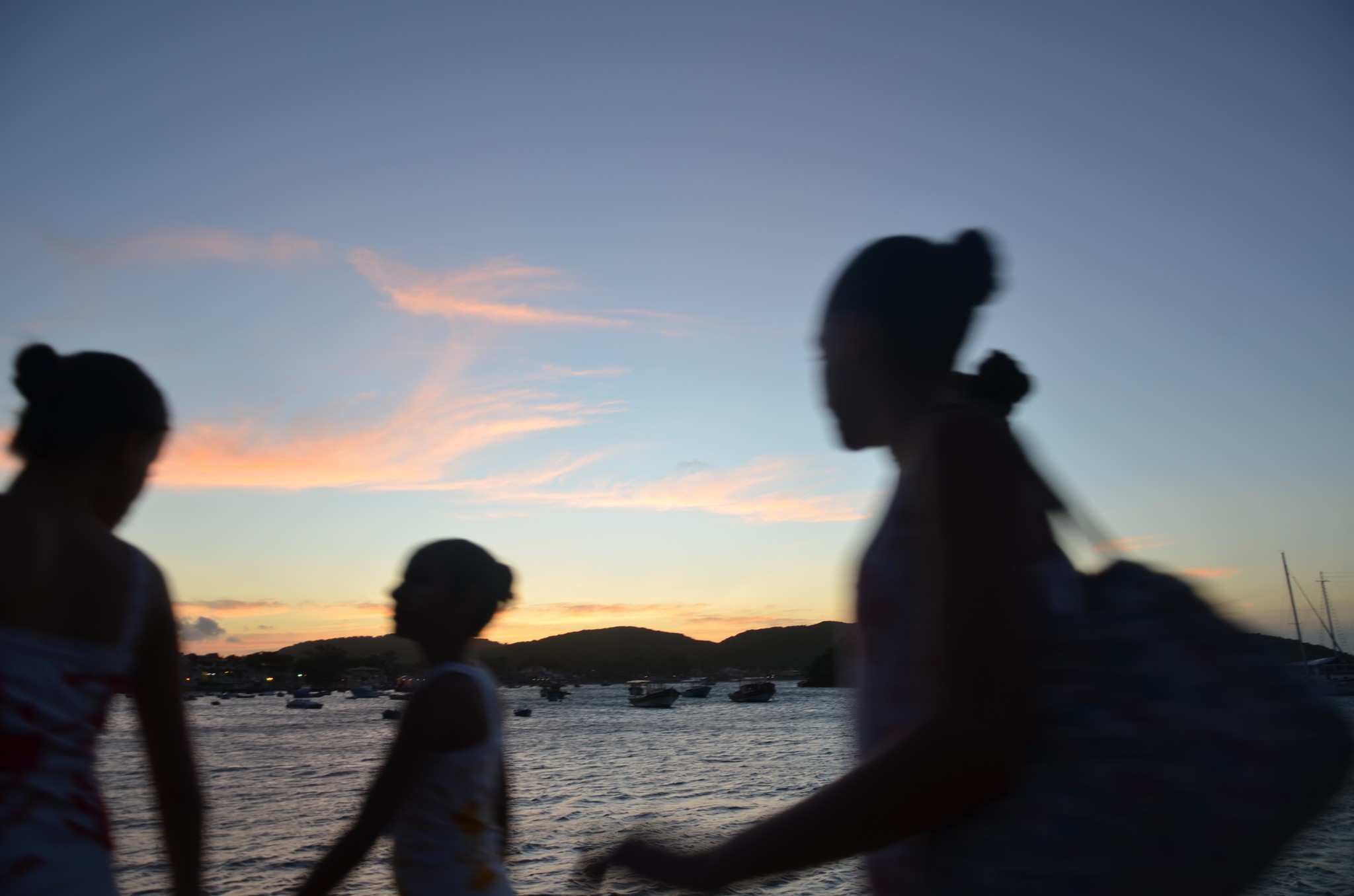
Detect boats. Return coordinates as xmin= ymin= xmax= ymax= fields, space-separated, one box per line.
xmin=389 ymin=693 xmax=411 ymax=700
xmin=381 ymin=709 xmax=402 ymax=719
xmin=180 ymin=694 xmax=256 ymax=700
xmin=513 ymin=708 xmax=532 ymax=717
xmin=540 ymin=683 xmax=572 ymax=701
xmin=277 ymin=692 xmax=285 ymax=697
xmin=285 ymin=699 xmax=323 ymax=709
xmin=727 ymin=677 xmax=776 ymax=703
xmin=259 ymin=693 xmax=277 ymax=696
xmin=293 ymin=688 xmax=331 ymax=698
xmin=626 ymin=680 xmax=680 ymax=708
xmin=211 ymin=701 xmax=221 ymax=705
xmin=678 ymin=679 xmax=712 ymax=698
xmin=350 ymin=688 xmax=381 ymax=698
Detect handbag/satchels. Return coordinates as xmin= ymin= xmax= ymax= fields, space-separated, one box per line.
xmin=867 ymin=542 xmax=1353 ymax=896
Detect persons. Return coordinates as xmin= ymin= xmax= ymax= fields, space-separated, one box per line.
xmin=566 ymin=228 xmax=1072 ymax=895
xmin=292 ymin=538 xmax=520 ymax=896
xmin=0 ymin=343 xmax=211 ymax=896
